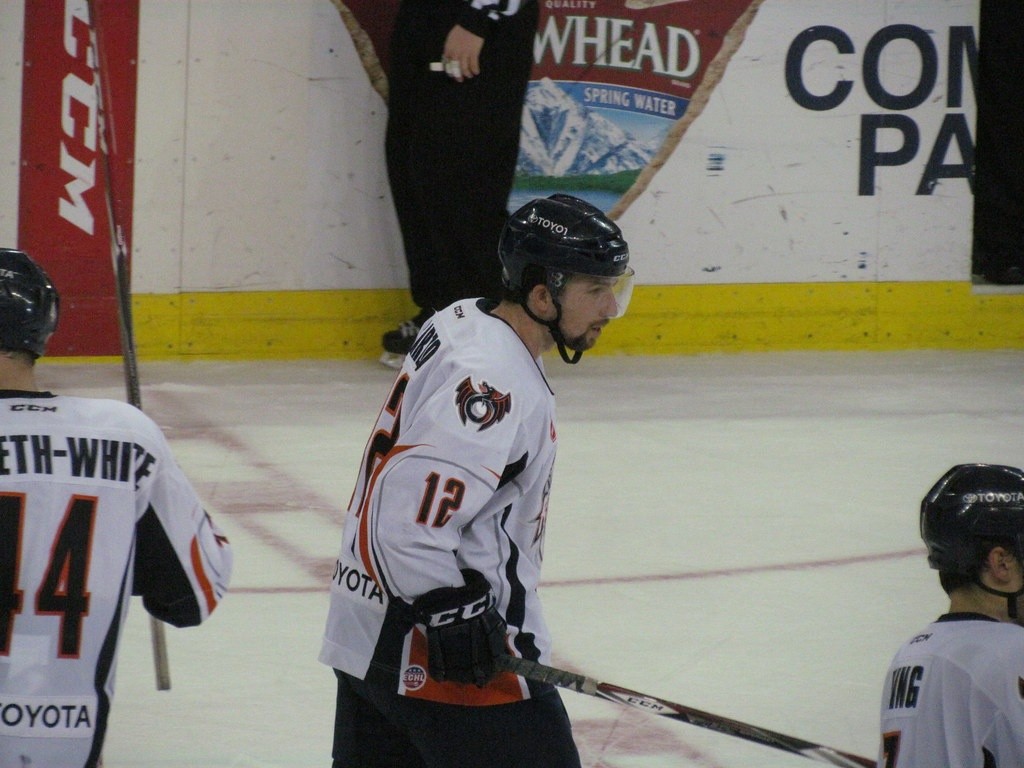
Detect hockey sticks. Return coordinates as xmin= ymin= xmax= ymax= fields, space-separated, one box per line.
xmin=491 ymin=653 xmax=880 ymax=768
xmin=90 ymin=8 xmax=173 ymax=692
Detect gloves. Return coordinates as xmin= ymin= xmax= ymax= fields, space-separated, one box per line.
xmin=415 ymin=568 xmax=510 ymax=687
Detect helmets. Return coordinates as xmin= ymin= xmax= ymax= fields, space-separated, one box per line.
xmin=919 ymin=462 xmax=1024 ymax=573
xmin=0 ymin=248 xmax=59 ymax=358
xmin=498 ymin=193 xmax=630 ymax=296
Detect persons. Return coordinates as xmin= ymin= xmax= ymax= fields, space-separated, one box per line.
xmin=319 ymin=193 xmax=635 ymax=768
xmin=0 ymin=247 xmax=233 ymax=766
xmin=381 ymin=0 xmax=540 ymax=356
xmin=876 ymin=462 xmax=1024 ymax=768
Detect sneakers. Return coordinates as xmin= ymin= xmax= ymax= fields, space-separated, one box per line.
xmin=378 ymin=306 xmax=438 ymax=369
